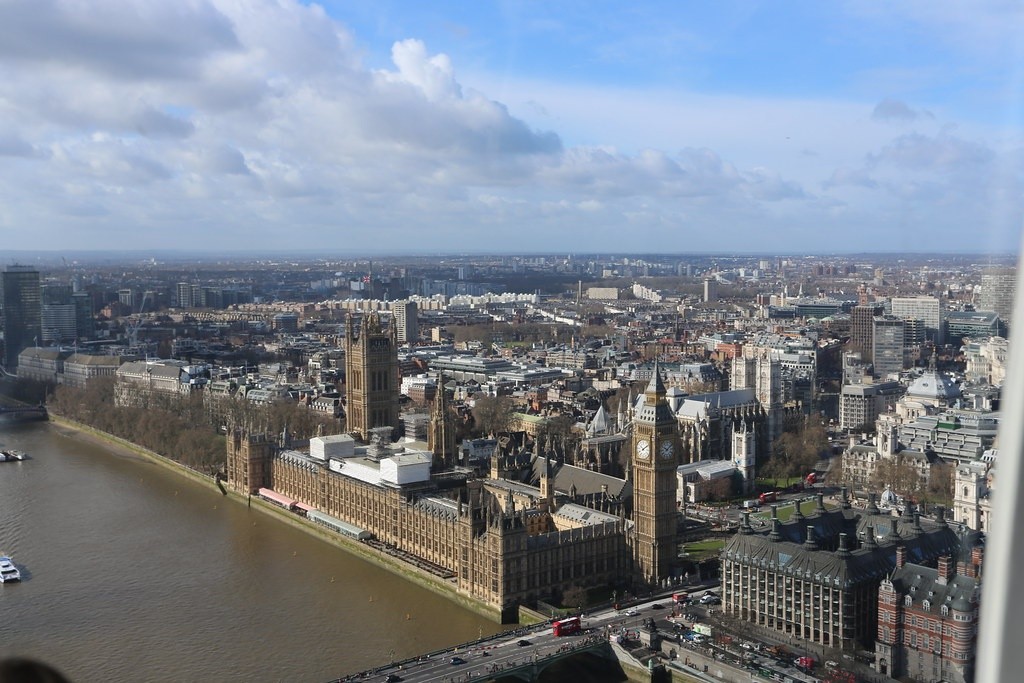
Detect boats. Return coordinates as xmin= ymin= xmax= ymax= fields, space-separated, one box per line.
xmin=1 ymin=550 xmax=21 ymax=585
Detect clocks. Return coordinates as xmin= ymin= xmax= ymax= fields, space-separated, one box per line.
xmin=660 ymin=440 xmax=675 ymax=460
xmin=636 ymin=440 xmax=650 ymax=460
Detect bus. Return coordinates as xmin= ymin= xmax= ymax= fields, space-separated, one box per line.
xmin=551 ymin=616 xmax=581 ymax=637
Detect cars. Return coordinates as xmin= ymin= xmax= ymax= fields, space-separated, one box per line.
xmin=386 ymin=592 xmax=855 ymax=683
